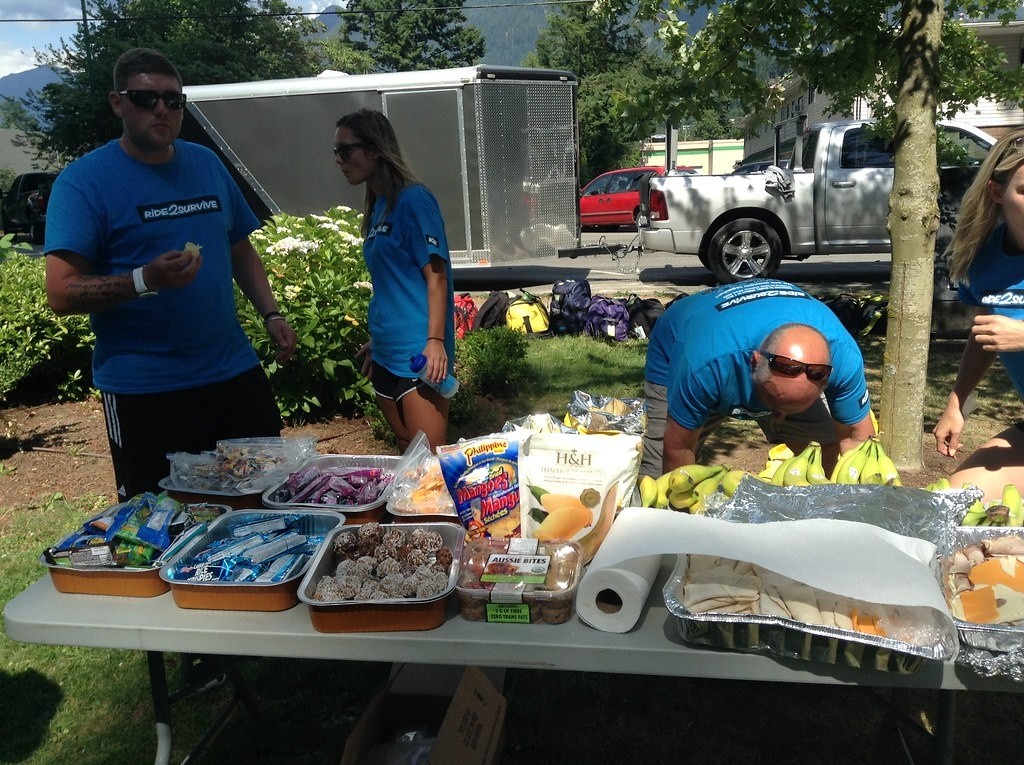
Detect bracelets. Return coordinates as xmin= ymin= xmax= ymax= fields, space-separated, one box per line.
xmin=263 ymin=311 xmax=282 ymax=320
xmin=427 ymin=337 xmax=444 ymax=342
xmin=266 ymin=316 xmax=286 ymax=324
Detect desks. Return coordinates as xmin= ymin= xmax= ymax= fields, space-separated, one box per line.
xmin=1 ymin=493 xmax=1024 ymax=765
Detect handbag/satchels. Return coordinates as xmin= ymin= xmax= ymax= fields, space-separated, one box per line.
xmin=583 ymin=294 xmax=629 ymax=341
xmin=812 ymin=294 xmax=861 ymax=336
xmin=859 ymin=291 xmax=888 ymax=334
xmin=505 ymin=289 xmax=549 ymax=334
xmin=625 ymin=293 xmax=665 ymax=339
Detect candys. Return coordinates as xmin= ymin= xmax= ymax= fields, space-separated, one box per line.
xmin=41 ymin=440 xmax=398 ymax=583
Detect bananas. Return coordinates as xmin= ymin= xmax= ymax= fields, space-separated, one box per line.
xmin=636 ymin=435 xmax=905 ymax=513
xmin=926 ymin=478 xmax=1024 ymax=531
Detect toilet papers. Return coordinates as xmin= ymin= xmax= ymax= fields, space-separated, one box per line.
xmin=577 ymin=504 xmax=668 ymax=634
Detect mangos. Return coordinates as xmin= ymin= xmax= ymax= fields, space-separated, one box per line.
xmin=532 ymin=493 xmax=593 ymax=542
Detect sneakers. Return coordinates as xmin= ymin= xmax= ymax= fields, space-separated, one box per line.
xmin=181 ymin=653 xmax=226 ymax=696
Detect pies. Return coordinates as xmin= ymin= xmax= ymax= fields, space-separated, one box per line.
xmin=683 ymin=555 xmax=893 ymax=640
xmin=946 ymin=536 xmax=1024 ymax=628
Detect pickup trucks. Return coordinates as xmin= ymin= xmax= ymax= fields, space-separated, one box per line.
xmin=0 ymin=172 xmax=61 ymax=243
xmin=635 ymin=113 xmax=1000 ymax=286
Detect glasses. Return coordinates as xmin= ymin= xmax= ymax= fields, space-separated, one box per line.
xmin=758 ymin=350 xmax=832 ymax=380
xmin=991 ymin=135 xmax=1024 ymax=176
xmin=334 ymin=143 xmax=368 ymax=159
xmin=119 ymin=90 xmax=186 ymax=109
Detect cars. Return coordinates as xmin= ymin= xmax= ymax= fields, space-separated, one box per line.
xmin=576 ymin=164 xmax=704 ymax=225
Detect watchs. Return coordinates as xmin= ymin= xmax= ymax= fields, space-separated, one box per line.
xmin=131 ymin=264 xmax=160 ymax=296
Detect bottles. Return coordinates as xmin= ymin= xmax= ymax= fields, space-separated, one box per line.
xmin=410 ymin=354 xmax=460 ymax=398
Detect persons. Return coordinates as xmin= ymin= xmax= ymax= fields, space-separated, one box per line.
xmin=332 ymin=106 xmax=456 ymax=459
xmin=45 ymin=49 xmax=297 ymax=692
xmin=931 ymin=128 xmax=1024 ymax=503
xmin=636 ymin=277 xmax=876 ymax=476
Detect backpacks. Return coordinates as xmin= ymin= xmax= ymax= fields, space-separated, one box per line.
xmin=549 ymin=280 xmax=591 ymax=334
xmin=454 ymin=293 xmax=477 ymax=338
xmin=471 ymin=292 xmax=510 ymax=331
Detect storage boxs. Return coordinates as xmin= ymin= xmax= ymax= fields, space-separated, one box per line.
xmin=299 ymin=525 xmax=462 ymax=631
xmin=263 ymin=454 xmax=396 ymax=519
xmin=341 ymin=661 xmax=509 ymax=765
xmin=455 ymin=537 xmax=584 ymax=627
xmin=386 ymin=450 xmax=466 ymax=518
xmin=39 ymin=502 xmax=232 ymax=597
xmin=157 ymin=509 xmax=344 ymax=611
xmin=156 ymin=437 xmax=307 ymax=508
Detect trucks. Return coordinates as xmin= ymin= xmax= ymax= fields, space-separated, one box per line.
xmin=176 ymin=66 xmax=583 ymax=270
